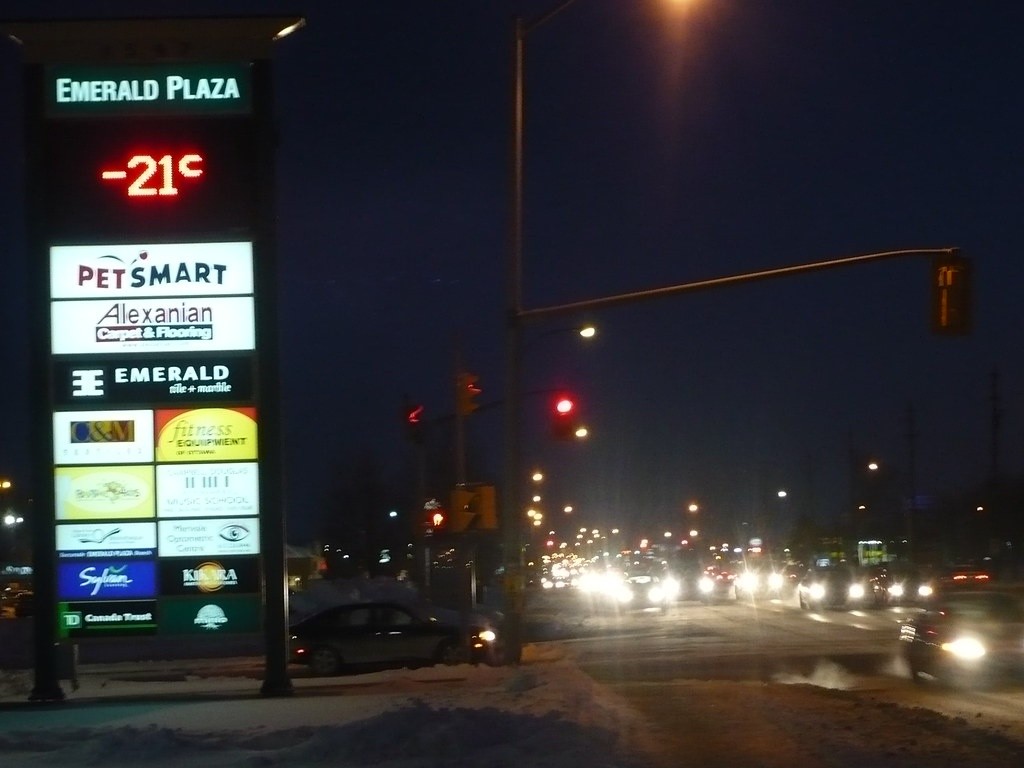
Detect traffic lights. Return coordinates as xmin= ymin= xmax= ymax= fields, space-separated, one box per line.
xmin=557 ymin=393 xmax=580 ymax=419
xmin=935 ymin=262 xmax=976 ymax=342
xmin=430 ymin=509 xmax=446 ymax=528
xmin=404 ymin=403 xmax=426 ymax=425
xmin=451 ymin=483 xmax=499 ymax=531
xmin=444 ymin=367 xmax=481 ymax=401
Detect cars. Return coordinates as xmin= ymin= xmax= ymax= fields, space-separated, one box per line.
xmin=569 ymin=549 xmax=793 ymax=610
xmin=798 ymin=568 xmax=867 ymax=611
xmin=901 ymin=590 xmax=1024 ymax=693
xmin=939 ymin=564 xmax=989 ymax=589
xmin=877 ymin=563 xmax=933 ymax=604
xmin=288 ymin=601 xmax=523 ymax=673
xmin=829 ymin=559 xmax=848 ymax=569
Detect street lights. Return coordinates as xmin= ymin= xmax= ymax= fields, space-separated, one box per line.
xmin=772 ymin=487 xmax=802 ymax=538
xmin=512 ymin=423 xmax=587 ymax=636
xmin=868 ymin=458 xmax=918 ymax=583
xmin=506 ymin=322 xmax=598 ymax=657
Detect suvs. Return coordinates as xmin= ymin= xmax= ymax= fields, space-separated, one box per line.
xmin=814 ymin=556 xmax=832 ymax=570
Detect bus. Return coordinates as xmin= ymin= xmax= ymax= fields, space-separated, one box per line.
xmin=847 ymin=540 xmax=887 ymax=568
